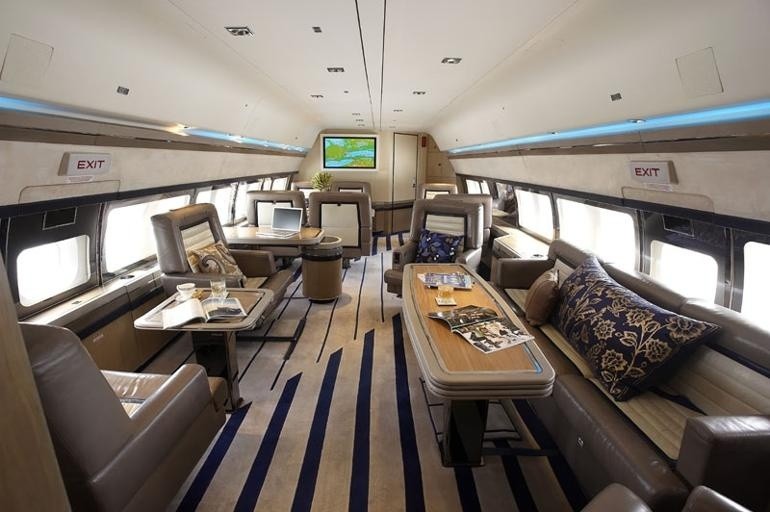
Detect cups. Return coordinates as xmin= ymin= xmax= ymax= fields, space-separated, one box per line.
xmin=438 ymin=285 xmax=454 ymax=301
xmin=176 ymin=282 xmax=196 ymax=296
xmin=210 ymin=279 xmax=226 ymax=298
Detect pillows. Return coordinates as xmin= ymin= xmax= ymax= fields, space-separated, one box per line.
xmin=525 ymin=257 xmax=560 ymax=326
xmin=552 ymin=253 xmax=723 ymax=402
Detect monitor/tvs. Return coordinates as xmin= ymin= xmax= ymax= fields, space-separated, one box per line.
xmin=320 ymin=134 xmax=379 ymax=172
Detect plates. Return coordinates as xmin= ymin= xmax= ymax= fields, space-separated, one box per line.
xmin=174 ymin=296 xmax=193 ymax=301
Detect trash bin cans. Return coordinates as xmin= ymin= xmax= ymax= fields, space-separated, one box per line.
xmin=300 ymin=235 xmax=342 ymax=304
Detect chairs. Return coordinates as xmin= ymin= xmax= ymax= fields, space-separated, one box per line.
xmin=580 ymin=483 xmax=751 ymax=511
xmin=18 ymin=322 xmax=229 ymax=510
xmin=384 ymin=183 xmax=493 ymax=294
xmin=150 ymin=180 xmax=376 ymax=343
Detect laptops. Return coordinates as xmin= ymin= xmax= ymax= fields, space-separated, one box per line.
xmin=255 ymin=207 xmax=303 ymax=239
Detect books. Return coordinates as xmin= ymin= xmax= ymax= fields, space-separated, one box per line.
xmin=416 ymin=270 xmax=474 ymax=289
xmin=425 ymin=303 xmax=534 ymax=354
xmin=160 ymin=295 xmax=248 ymax=330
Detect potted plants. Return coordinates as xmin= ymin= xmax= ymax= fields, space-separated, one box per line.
xmin=312 ymin=171 xmax=334 ymax=192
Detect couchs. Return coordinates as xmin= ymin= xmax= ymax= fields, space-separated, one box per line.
xmin=496 ymin=239 xmax=770 ymax=511
